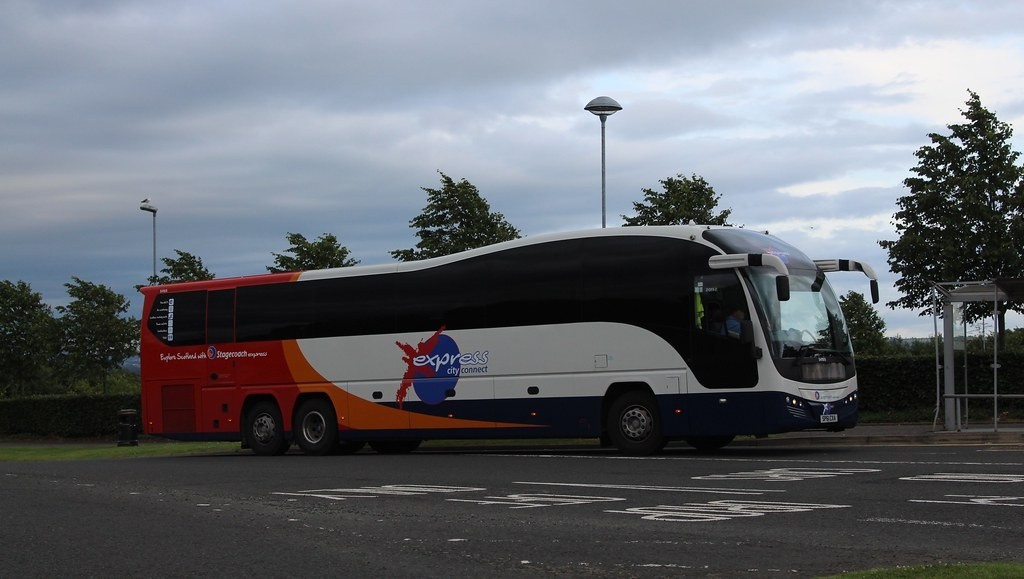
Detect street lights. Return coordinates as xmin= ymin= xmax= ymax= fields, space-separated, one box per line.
xmin=139 ymin=203 xmax=159 ymax=286
xmin=584 ymin=96 xmax=623 ymax=228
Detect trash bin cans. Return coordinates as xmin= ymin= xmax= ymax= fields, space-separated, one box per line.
xmin=117 ymin=409 xmax=138 ymax=447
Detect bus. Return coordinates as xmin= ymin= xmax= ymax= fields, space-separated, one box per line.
xmin=139 ymin=220 xmax=880 ymax=459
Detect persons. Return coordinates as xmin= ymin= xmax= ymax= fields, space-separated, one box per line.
xmin=721 ymin=309 xmax=745 ymax=338
